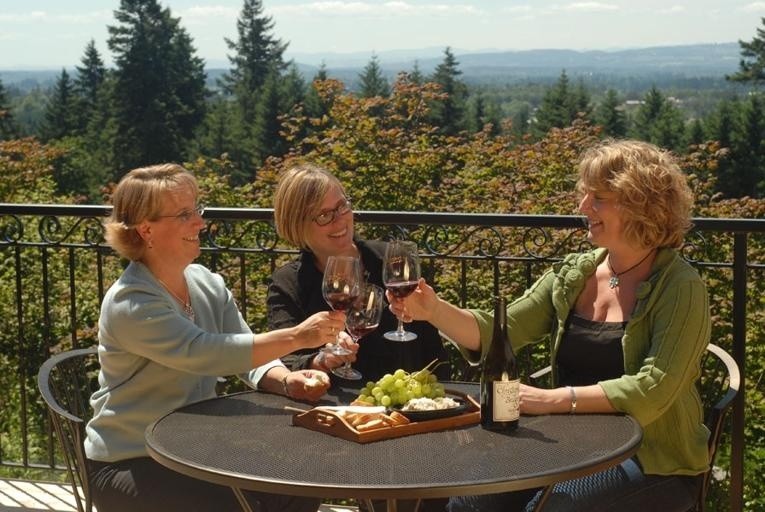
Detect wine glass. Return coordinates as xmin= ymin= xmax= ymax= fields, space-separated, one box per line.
xmin=381 ymin=239 xmax=421 ymax=342
xmin=330 ymin=280 xmax=384 ymax=380
xmin=321 ymin=254 xmax=363 ymax=355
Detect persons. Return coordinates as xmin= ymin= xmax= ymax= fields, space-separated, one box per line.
xmin=262 ymin=159 xmax=450 ymax=510
xmin=77 ymin=160 xmax=350 ymax=511
xmin=380 ymin=138 xmax=713 ymax=512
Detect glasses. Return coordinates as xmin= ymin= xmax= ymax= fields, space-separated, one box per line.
xmin=161 ymin=204 xmax=204 ymax=221
xmin=312 ymin=200 xmax=351 ymax=225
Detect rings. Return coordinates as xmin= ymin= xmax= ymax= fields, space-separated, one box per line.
xmin=329 ymin=327 xmax=335 ymax=334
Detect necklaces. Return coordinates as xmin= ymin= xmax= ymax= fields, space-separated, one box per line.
xmin=604 ymin=247 xmax=657 ymax=287
xmin=155 ymin=272 xmax=196 ymax=320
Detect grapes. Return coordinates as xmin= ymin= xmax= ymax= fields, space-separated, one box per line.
xmin=358 ymin=369 xmax=445 ymax=406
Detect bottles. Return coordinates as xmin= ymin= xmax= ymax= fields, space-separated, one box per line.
xmin=480 ymin=295 xmax=520 ymax=432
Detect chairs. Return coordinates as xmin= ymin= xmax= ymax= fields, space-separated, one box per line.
xmin=529 ymin=337 xmax=742 ymax=510
xmin=36 ymin=343 xmax=227 ymax=510
xmin=430 ymin=325 xmax=481 ymax=384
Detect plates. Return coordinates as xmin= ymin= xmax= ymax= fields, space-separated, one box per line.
xmin=388 ymin=392 xmax=467 ymax=419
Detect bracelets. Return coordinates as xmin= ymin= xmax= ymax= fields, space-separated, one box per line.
xmin=316 ymin=349 xmax=329 ymax=368
xmin=564 ymin=384 xmax=577 ymax=415
xmin=281 ymin=372 xmax=291 ymax=397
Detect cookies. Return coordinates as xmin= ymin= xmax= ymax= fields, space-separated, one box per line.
xmin=345 ymin=401 xmax=409 ymax=431
